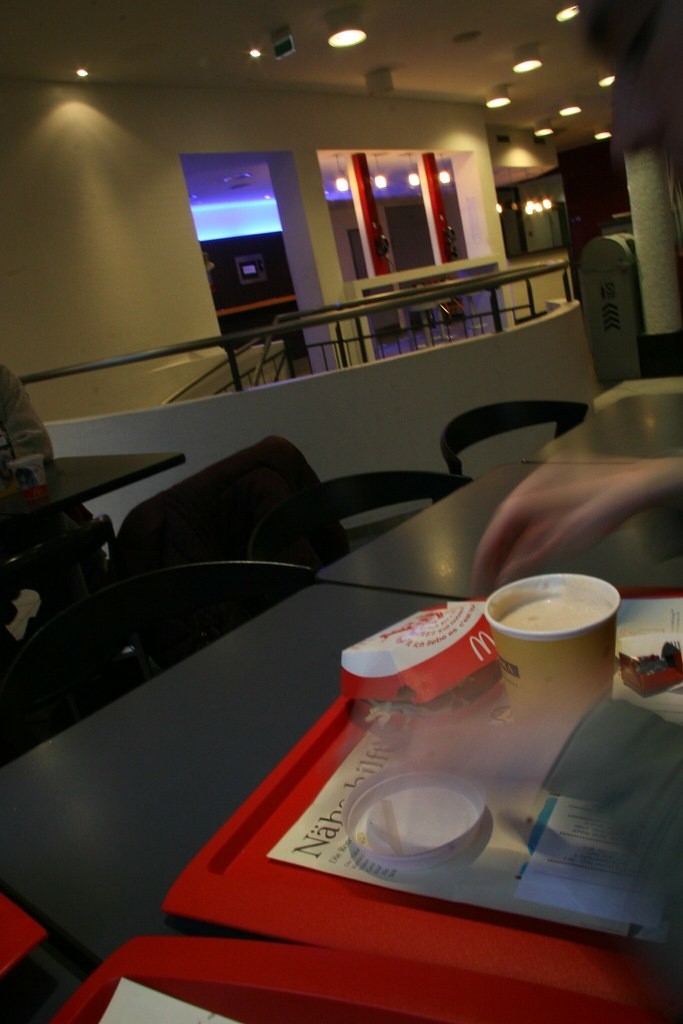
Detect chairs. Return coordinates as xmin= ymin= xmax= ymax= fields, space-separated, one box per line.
xmin=119 ymin=435 xmax=348 ymax=674
xmin=247 ymin=471 xmax=473 ymax=568
xmin=0 ymin=514 xmax=149 ymax=771
xmin=0 ymin=560 xmax=306 ymax=765
xmin=439 ymin=400 xmax=588 ymax=477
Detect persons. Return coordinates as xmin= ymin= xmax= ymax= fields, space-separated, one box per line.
xmin=468 ymin=455 xmax=681 ymax=586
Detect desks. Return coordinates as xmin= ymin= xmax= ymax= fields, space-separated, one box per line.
xmin=0 ymin=382 xmax=683 ymax=1024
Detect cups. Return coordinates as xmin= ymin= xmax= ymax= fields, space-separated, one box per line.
xmin=484 ymin=574 xmax=622 ymax=750
xmin=8 ymin=454 xmax=48 ymax=504
